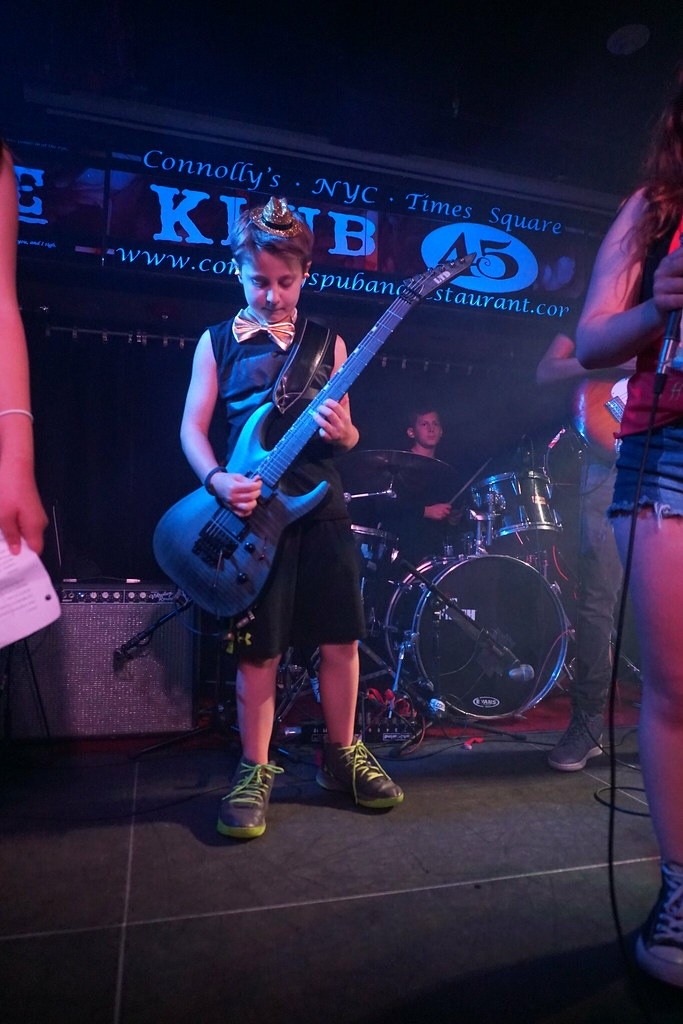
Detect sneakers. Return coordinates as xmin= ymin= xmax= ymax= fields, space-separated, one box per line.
xmin=636 ymin=861 xmax=683 ymax=986
xmin=315 ymin=737 xmax=404 ymax=808
xmin=547 ymin=710 xmax=608 ymax=770
xmin=218 ymin=756 xmax=285 ymax=836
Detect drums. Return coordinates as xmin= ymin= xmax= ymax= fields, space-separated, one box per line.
xmin=469 ymin=471 xmax=564 ymax=556
xmin=387 ymin=553 xmax=571 ymax=715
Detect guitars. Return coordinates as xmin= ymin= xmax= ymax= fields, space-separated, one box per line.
xmin=152 ymin=250 xmax=480 ymax=619
xmin=566 ymin=365 xmax=634 ymax=462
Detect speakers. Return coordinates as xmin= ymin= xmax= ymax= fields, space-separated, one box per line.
xmin=6 ymin=581 xmax=200 ymax=737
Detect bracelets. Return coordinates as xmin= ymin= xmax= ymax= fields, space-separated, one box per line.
xmin=205 ymin=466 xmax=227 ymax=496
xmin=0 ymin=409 xmax=33 ymax=421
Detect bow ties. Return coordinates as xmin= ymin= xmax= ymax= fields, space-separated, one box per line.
xmin=232 ymin=308 xmax=296 ymax=351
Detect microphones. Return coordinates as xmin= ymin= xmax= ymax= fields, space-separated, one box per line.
xmin=114 ymin=648 xmax=129 ymax=663
xmin=509 ymin=666 xmax=535 ymax=684
xmin=517 ymin=436 xmax=530 ymax=473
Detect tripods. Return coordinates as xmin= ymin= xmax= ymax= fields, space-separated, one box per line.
xmin=389 ymin=560 xmax=527 ymax=759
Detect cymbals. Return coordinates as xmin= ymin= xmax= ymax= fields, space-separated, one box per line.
xmin=359 ymin=448 xmax=450 ymax=480
xmin=349 ymin=519 xmax=399 ymax=542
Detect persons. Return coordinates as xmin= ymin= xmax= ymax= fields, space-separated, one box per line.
xmin=573 ymin=96 xmax=683 ymax=990
xmin=179 ymin=203 xmax=403 ymax=839
xmin=534 ymin=279 xmax=637 ymax=771
xmin=0 ymin=146 xmax=51 ymax=556
xmin=394 ymin=406 xmax=468 ymax=562
